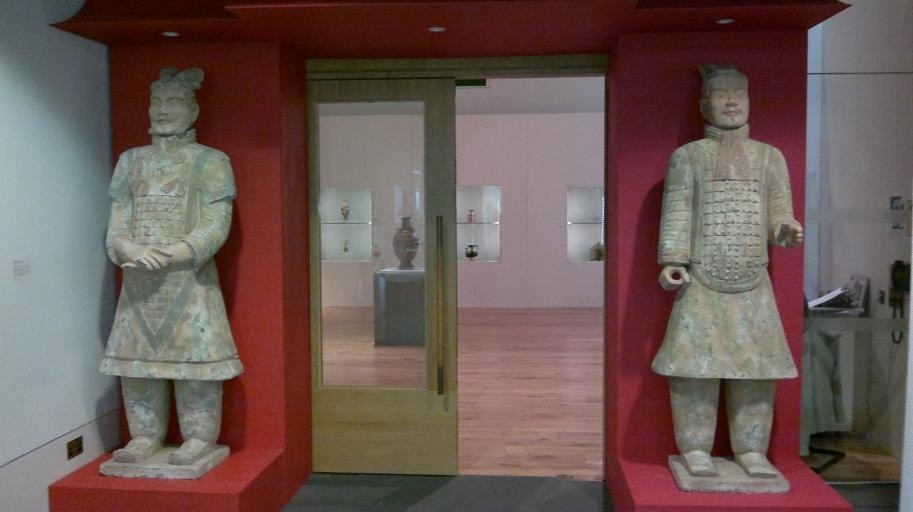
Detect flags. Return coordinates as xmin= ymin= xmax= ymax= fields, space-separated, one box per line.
xmin=392 ymin=216 xmax=418 ymax=269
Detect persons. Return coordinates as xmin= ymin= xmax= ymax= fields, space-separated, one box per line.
xmin=651 ymin=64 xmax=804 ymax=481
xmin=97 ymin=66 xmax=237 ymax=467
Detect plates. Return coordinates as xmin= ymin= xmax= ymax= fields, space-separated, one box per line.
xmin=454 ymin=184 xmax=500 ymax=263
xmin=564 ymin=185 xmax=605 ymax=263
xmin=320 ymin=186 xmax=373 ymax=260
